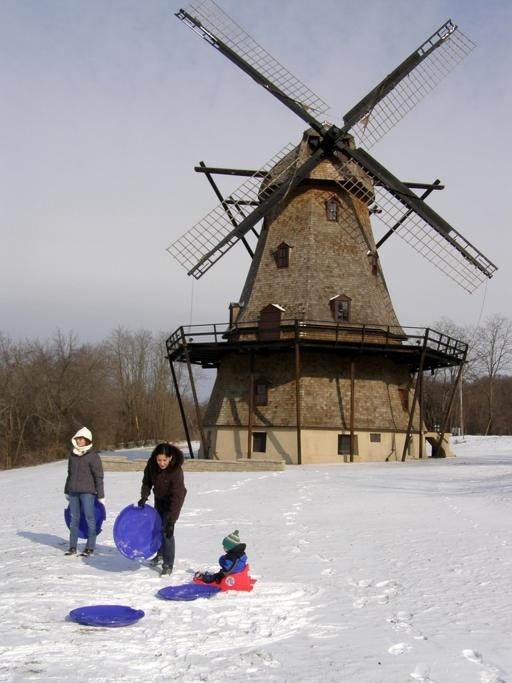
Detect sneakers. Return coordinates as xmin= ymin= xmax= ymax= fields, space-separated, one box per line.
xmin=79 ymin=548 xmax=93 ymax=556
xmin=64 ymin=547 xmax=76 ymax=555
xmin=151 ymin=552 xmax=163 ymax=562
xmin=161 ymin=564 xmax=172 ymax=577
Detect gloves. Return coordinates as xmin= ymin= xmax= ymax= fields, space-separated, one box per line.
xmin=138 ymin=498 xmax=146 ymax=506
xmin=165 ymin=523 xmax=174 ymax=538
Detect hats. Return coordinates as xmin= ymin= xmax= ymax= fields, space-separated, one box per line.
xmin=222 ymin=530 xmax=240 ymax=549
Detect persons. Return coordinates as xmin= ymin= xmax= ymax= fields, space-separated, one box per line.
xmin=192 ymin=528 xmax=248 ymax=585
xmin=63 ymin=425 xmax=106 ymax=557
xmin=136 ymin=440 xmax=188 ymax=576
